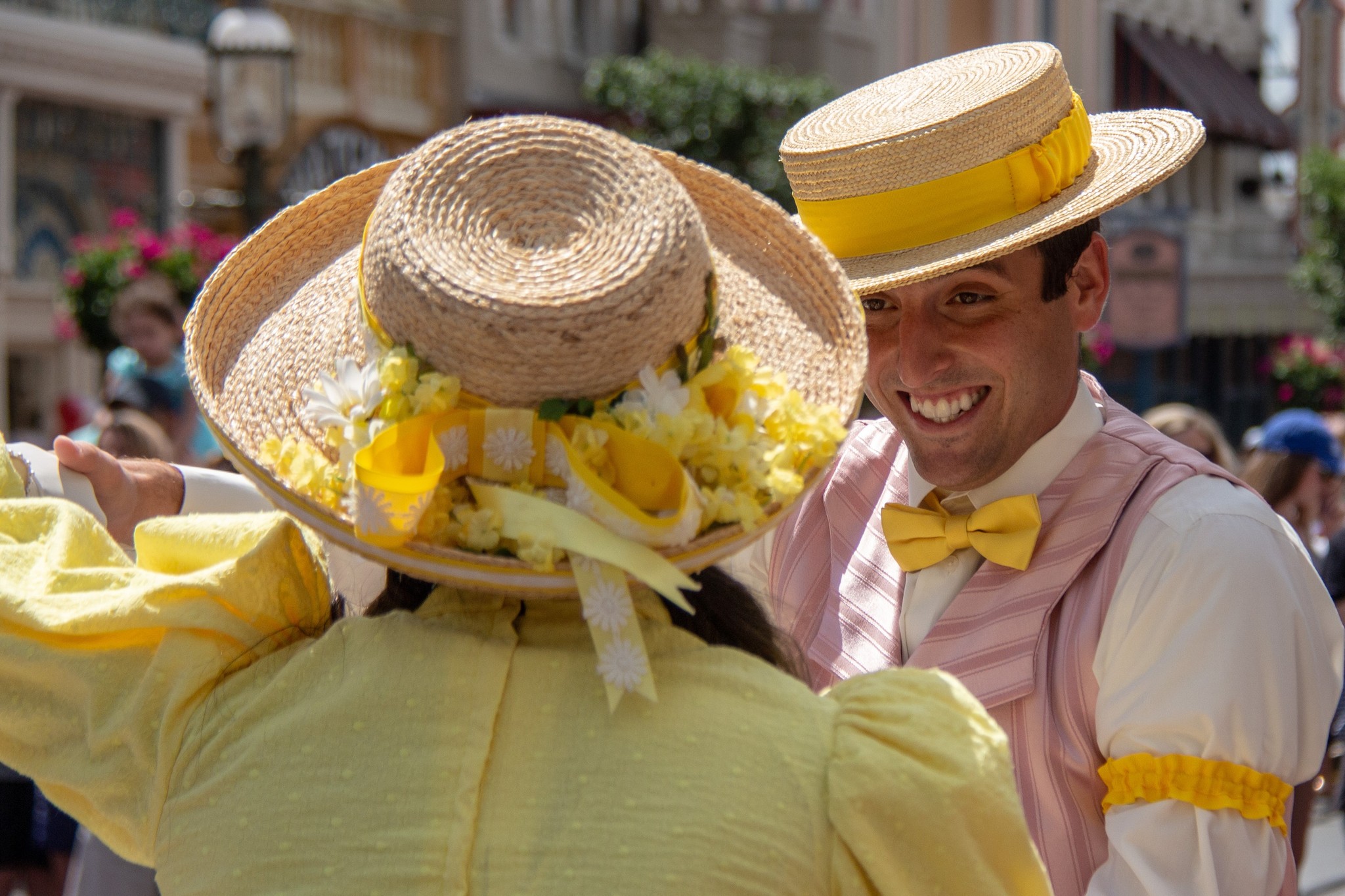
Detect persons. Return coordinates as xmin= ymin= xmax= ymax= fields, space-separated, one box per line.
xmin=0 ymin=284 xmax=238 ymax=896
xmin=0 ymin=117 xmax=1057 ymax=896
xmin=1145 ymin=404 xmax=1345 ymax=896
xmin=14 ymin=41 xmax=1345 ymax=896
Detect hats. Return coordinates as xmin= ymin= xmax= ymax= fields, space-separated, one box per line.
xmin=182 ymin=111 xmax=870 ymax=712
xmin=1252 ymin=410 xmax=1342 ymax=479
xmin=779 ymin=39 xmax=1208 ymax=295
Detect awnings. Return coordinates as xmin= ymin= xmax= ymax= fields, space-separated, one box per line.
xmin=1114 ymin=15 xmax=1288 ymax=149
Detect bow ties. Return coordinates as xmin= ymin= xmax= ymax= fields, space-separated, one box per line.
xmin=880 ymin=493 xmax=1042 ymax=574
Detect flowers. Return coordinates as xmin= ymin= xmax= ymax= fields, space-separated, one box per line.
xmin=257 ymin=339 xmax=848 ymax=714
xmin=1266 ymin=325 xmax=1344 ymax=409
xmin=56 ymin=207 xmax=241 ymax=341
